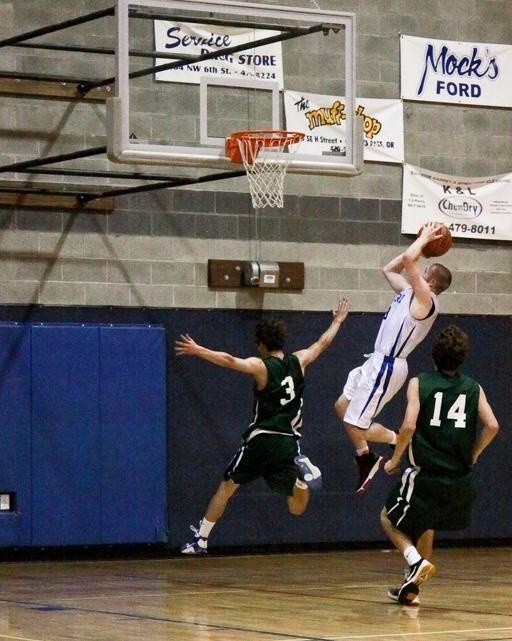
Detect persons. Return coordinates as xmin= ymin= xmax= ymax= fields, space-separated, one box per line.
xmin=171 ymin=296 xmax=351 ymax=556
xmin=379 ymin=324 xmax=500 ymax=608
xmin=332 ymin=220 xmax=452 ymax=498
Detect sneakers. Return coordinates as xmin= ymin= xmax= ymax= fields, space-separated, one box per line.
xmin=398 ymin=558 xmax=435 ymax=605
xmin=294 ymin=455 xmax=322 ymax=491
xmin=354 ymin=449 xmax=386 ymax=495
xmin=181 ymin=533 xmax=208 ymax=554
xmin=387 ymin=587 xmax=421 ymax=604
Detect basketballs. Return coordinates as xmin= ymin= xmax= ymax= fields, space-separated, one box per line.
xmin=417 ymin=222 xmax=452 ymax=257
